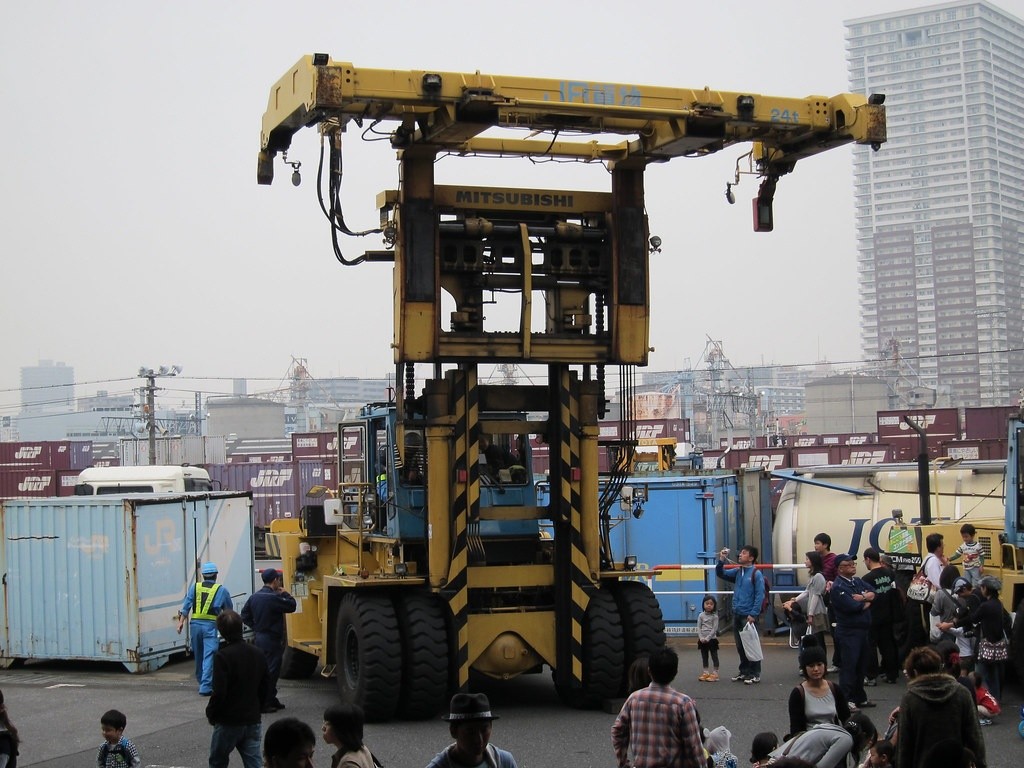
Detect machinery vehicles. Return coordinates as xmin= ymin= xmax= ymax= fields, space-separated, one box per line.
xmin=255 ymin=51 xmax=883 ymax=714
xmin=886 ymin=411 xmax=1024 ymax=690
xmin=609 ymin=438 xmax=679 ymax=473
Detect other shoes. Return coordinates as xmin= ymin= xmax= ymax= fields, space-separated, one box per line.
xmin=799 ymin=670 xmax=804 ymax=675
xmin=262 ymin=697 xmax=285 ymax=713
xmin=979 ymin=718 xmax=993 ymax=726
xmin=199 ymin=691 xmax=213 ymax=696
xmin=825 ymin=665 xmax=840 ymax=673
xmin=847 ymin=672 xmax=894 ymax=713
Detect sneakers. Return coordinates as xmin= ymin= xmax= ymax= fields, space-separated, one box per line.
xmin=731 ymin=671 xmax=751 ymax=681
xmin=706 ymin=674 xmax=720 ymax=682
xmin=744 ymin=674 xmax=760 ymax=685
xmin=698 ymin=673 xmax=710 ymax=681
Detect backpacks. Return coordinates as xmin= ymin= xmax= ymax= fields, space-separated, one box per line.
xmin=735 ymin=566 xmax=770 ymax=614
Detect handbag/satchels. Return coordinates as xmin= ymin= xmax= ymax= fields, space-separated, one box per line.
xmin=977 ymin=638 xmax=1009 ymax=661
xmin=906 ymin=573 xmax=936 ymax=604
xmin=929 ymin=611 xmax=942 ymax=643
xmin=739 ymin=621 xmax=764 ymax=661
xmin=801 ymin=624 xmax=812 ymax=650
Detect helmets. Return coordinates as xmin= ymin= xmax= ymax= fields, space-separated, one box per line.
xmin=201 ymin=561 xmax=219 ymax=574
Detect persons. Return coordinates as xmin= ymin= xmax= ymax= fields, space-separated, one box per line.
xmin=697 ymin=596 xmax=720 ymax=682
xmin=321 ymin=703 xmax=384 ymax=768
xmin=0 ymin=689 xmax=19 ymax=768
xmin=715 ymin=546 xmax=766 ymax=685
xmin=426 ymin=694 xmax=521 ymax=768
xmin=205 ymin=611 xmax=266 ymax=768
xmin=96 ymin=709 xmax=141 ymax=768
xmin=611 ymin=524 xmax=1024 ymax=768
xmin=264 ymin=717 xmax=316 ymax=768
xmin=177 ymin=563 xmax=233 ymax=696
xmin=241 ymin=569 xmax=297 ymax=713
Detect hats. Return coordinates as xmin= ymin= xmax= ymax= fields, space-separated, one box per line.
xmin=441 ymin=692 xmax=501 ymax=722
xmin=978 ymin=575 xmax=1002 ymax=590
xmin=261 ymin=568 xmax=283 ymax=582
xmin=834 ymin=554 xmax=858 ymax=568
xmin=881 ymin=555 xmax=894 ymax=569
xmin=801 ymin=635 xmax=827 ymax=680
xmin=703 ymin=726 xmax=731 ymax=753
xmin=950 ymin=576 xmax=969 ymax=596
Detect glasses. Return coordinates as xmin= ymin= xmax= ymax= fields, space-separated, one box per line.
xmin=322 ymin=719 xmax=331 ymax=725
xmin=838 ymin=563 xmax=857 ymax=567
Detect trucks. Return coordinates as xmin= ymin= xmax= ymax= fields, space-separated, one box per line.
xmin=75 ymin=467 xmax=222 ymax=494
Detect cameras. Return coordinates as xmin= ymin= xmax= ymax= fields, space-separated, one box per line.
xmin=724 ymin=548 xmax=730 ymax=554
